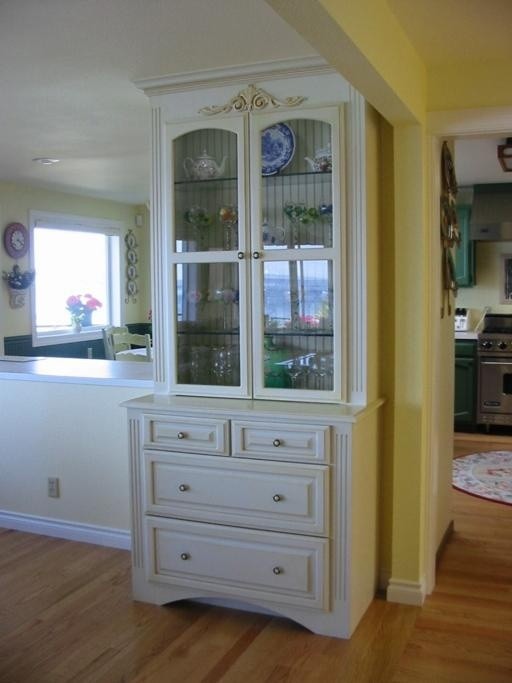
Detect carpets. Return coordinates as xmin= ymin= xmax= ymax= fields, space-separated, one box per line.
xmin=450 ymin=449 xmax=510 ymax=507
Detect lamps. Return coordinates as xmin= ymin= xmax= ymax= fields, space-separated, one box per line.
xmin=495 ymin=136 xmax=512 ymax=172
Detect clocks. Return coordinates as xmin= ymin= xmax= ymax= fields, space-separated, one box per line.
xmin=5 ymin=222 xmax=29 ymax=260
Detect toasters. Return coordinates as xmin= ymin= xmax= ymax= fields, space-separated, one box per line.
xmin=453 ymin=305 xmax=469 ymax=329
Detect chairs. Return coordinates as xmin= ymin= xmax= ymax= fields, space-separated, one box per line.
xmin=99 ymin=323 xmax=153 ymax=362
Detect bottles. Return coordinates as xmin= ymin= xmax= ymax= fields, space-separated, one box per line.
xmin=261 ymin=335 xmax=294 ymax=388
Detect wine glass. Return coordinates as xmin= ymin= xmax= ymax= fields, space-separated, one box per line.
xmin=187 ymin=288 xmax=238 ymax=334
xmin=285 ymin=350 xmax=334 ymax=390
xmin=182 ymin=196 xmax=236 ymax=251
xmin=285 ymin=198 xmax=331 ymax=248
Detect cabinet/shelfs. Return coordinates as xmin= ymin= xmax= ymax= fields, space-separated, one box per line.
xmin=120 ymin=396 xmax=382 ymax=639
xmin=136 ymin=58 xmax=380 ymax=412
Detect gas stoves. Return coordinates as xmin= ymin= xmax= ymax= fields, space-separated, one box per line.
xmin=476 ymin=325 xmax=511 ymax=356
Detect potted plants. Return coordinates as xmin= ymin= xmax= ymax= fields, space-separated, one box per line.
xmin=72 ymin=316 xmax=81 ymax=329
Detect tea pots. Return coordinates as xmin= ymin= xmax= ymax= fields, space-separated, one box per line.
xmin=262 ymin=216 xmax=287 ymax=244
xmin=182 ymin=148 xmax=229 ymax=180
xmin=303 ymin=141 xmax=332 ymax=171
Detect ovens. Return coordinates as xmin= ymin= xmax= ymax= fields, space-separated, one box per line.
xmin=474 ymin=356 xmax=512 ymax=426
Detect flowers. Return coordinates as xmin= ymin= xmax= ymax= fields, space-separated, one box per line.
xmin=66 ymin=293 xmax=105 ymax=312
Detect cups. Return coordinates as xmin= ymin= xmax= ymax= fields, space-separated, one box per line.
xmin=188 ymin=342 xmax=238 ymax=384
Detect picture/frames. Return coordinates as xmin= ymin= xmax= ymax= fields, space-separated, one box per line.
xmin=498 ymin=252 xmax=511 ymax=304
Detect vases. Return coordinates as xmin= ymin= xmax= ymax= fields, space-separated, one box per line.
xmin=75 ymin=309 xmax=93 ymax=325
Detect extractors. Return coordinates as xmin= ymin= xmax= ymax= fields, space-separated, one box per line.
xmin=468 ymin=184 xmax=511 ymax=241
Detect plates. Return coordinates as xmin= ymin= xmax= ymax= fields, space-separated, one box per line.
xmin=261 ymin=121 xmax=294 ymax=175
